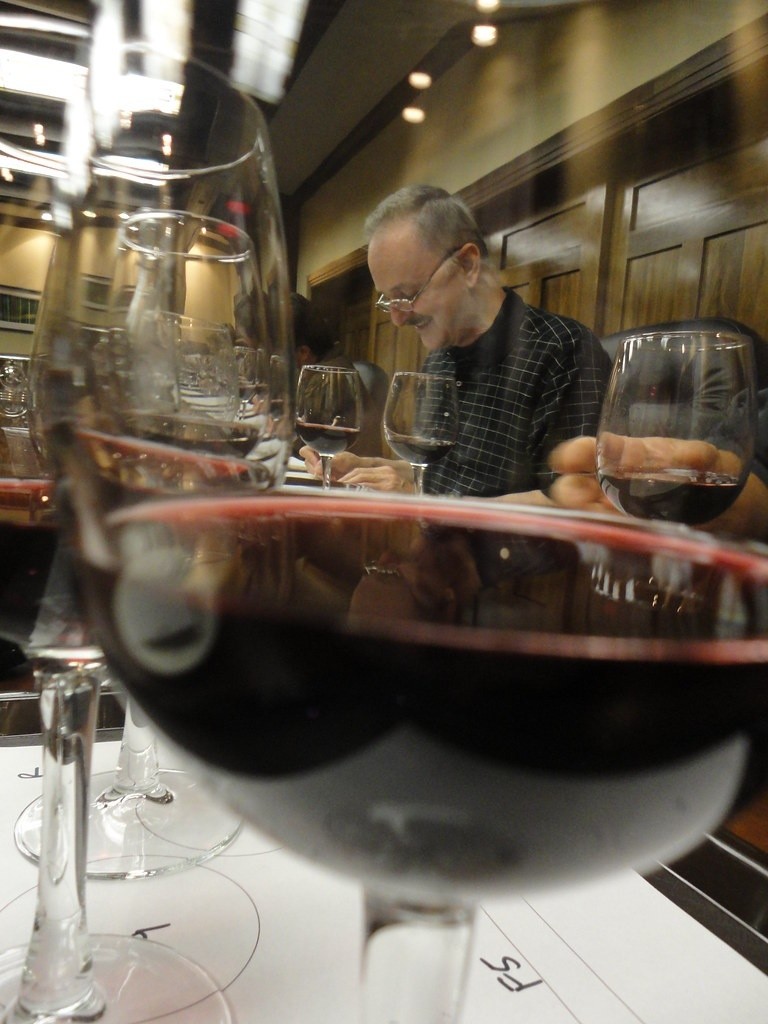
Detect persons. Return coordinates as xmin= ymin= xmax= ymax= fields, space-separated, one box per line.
xmin=294 ymin=183 xmax=614 ymax=632
xmin=173 ymin=285 xmax=384 ymax=461
xmin=544 ymin=383 xmax=768 ymax=543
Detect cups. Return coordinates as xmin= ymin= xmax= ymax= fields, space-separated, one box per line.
xmin=595 ymin=331 xmax=759 ymax=525
xmin=2 ymin=427 xmax=30 ymax=478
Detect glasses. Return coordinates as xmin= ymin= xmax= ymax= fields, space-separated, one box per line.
xmin=374 ymin=246 xmax=461 ymax=314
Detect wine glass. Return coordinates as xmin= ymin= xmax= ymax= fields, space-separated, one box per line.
xmin=383 ymin=371 xmax=460 ymax=496
xmin=295 ymin=365 xmax=364 ymax=491
xmin=32 ymin=0 xmax=768 ymax=1024
xmin=0 ymin=31 xmax=291 ymax=1024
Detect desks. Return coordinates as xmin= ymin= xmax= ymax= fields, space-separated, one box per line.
xmin=0 ymin=679 xmax=768 ymax=1023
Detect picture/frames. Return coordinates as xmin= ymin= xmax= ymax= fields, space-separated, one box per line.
xmin=0 ymin=285 xmax=42 ymax=331
xmin=83 ymin=272 xmax=113 ymax=311
